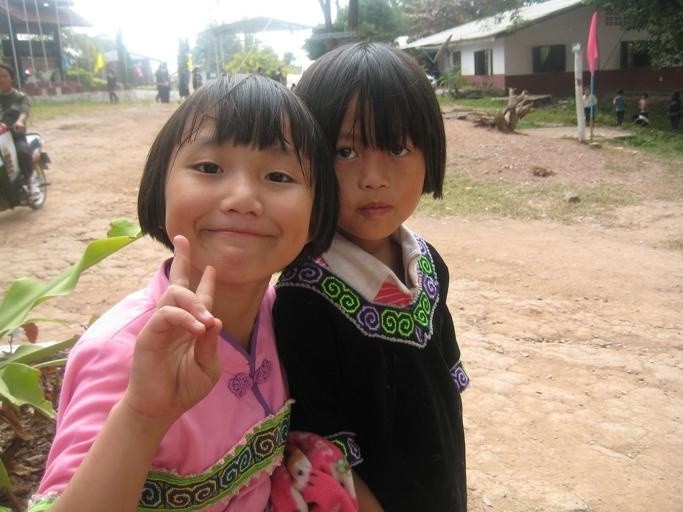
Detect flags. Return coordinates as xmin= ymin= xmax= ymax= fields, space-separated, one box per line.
xmin=58 ymin=38 xmax=153 ymax=81
xmin=586 ymin=12 xmax=598 ymax=77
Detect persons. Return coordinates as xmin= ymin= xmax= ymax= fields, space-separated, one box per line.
xmin=271 ymin=39 xmax=468 ymax=512
xmin=612 ymin=88 xmax=627 ymax=127
xmin=666 ymin=91 xmax=682 ymax=130
xmin=1 ymin=65 xmax=42 ymax=209
xmin=582 ymin=85 xmax=599 ymax=123
xmin=637 ymin=92 xmax=651 ymax=118
xmin=27 ymin=75 xmax=343 ymax=511
xmin=631 ymin=114 xmax=650 ymax=129
xmin=100 ymin=57 xmax=299 ymax=104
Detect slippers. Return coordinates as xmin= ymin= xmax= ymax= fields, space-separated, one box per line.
xmin=28 ymin=186 xmax=40 ymax=196
